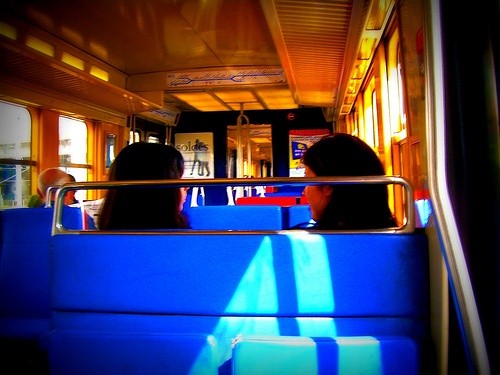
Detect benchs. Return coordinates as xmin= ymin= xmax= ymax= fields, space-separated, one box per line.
xmin=0 ymin=183 xmax=437 ymax=375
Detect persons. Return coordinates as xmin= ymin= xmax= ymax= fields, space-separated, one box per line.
xmin=36 ymin=169 xmax=98 ymax=230
xmin=198 ymin=142 xmax=210 ymax=176
xmin=289 ymin=133 xmax=400 ymax=231
xmin=97 ymin=142 xmax=191 ymax=230
xmin=190 ymin=138 xmax=202 ymax=176
